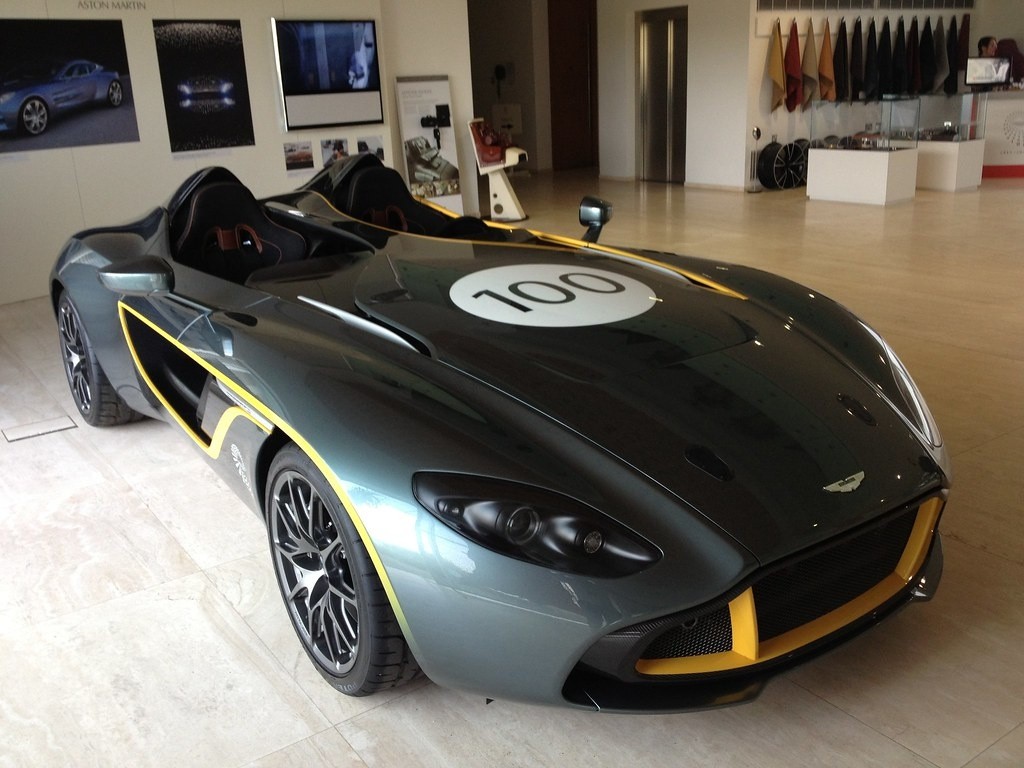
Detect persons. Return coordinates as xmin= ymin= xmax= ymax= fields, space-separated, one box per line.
xmin=433 ymin=125 xmax=442 ymax=150
xmin=969 ymin=37 xmax=1008 ymax=94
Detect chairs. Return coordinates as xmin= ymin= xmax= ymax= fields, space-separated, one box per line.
xmin=349 ymin=166 xmax=447 ymax=248
xmin=173 ymin=183 xmax=306 ymax=284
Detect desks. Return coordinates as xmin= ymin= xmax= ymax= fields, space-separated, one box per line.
xmin=960 ymin=90 xmax=1024 ymax=179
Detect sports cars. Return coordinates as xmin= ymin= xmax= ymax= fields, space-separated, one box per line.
xmin=0 ymin=60 xmax=126 ymax=138
xmin=47 ymin=150 xmax=955 ymax=718
